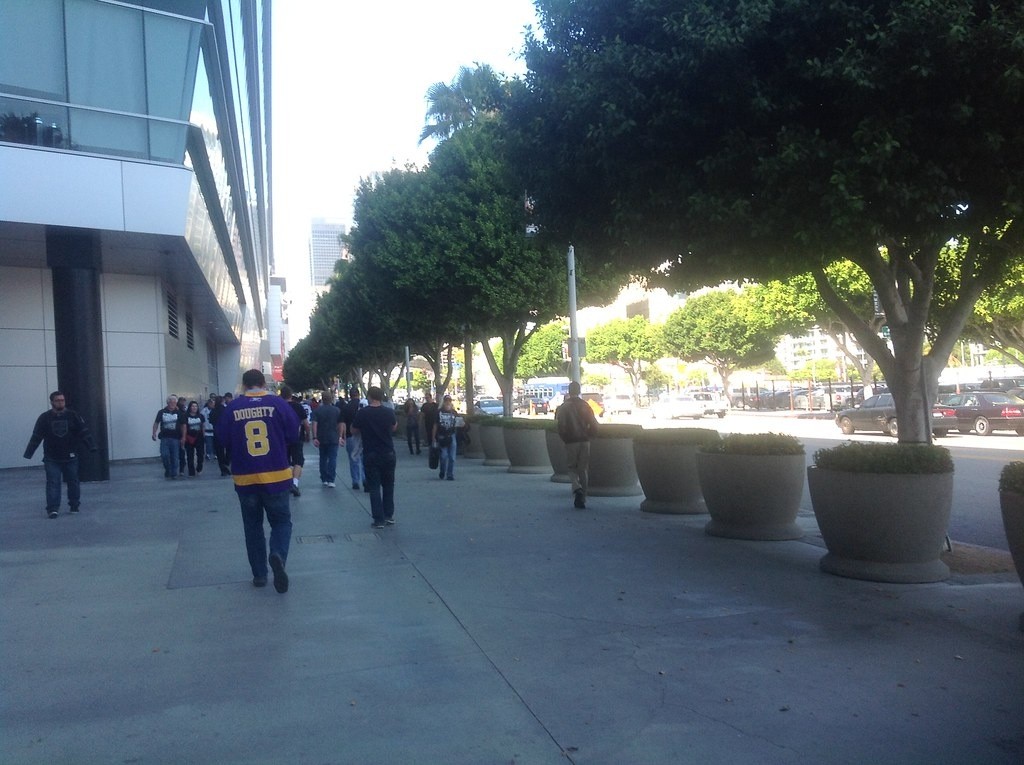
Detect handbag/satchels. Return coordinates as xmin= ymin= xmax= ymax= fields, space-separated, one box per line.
xmin=439 ymin=436 xmax=453 ymax=446
xmin=428 ymin=439 xmax=439 ymax=469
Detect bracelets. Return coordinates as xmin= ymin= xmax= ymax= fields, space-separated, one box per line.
xmin=313 ymin=437 xmax=317 ymax=440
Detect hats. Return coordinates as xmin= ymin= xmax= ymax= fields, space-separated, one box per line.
xmin=222 ymin=393 xmax=232 ymax=398
xmin=444 ymin=395 xmax=452 ymax=401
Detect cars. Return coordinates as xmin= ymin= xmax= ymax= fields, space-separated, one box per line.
xmin=941 ymin=391 xmax=1024 ymax=437
xmin=732 ymin=388 xmax=864 ymax=412
xmin=834 ymin=392 xmax=957 ymax=438
xmin=469 ymin=377 xmax=632 ymax=417
xmin=650 ymin=389 xmax=729 ymax=421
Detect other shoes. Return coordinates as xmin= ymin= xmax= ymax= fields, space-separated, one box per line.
xmin=70 ymin=507 xmax=79 ymax=513
xmin=447 ymin=474 xmax=453 ymax=480
xmin=384 ymin=518 xmax=396 ymax=524
xmin=253 ymin=577 xmax=268 ymax=587
xmin=163 ymin=464 xmax=235 ymax=480
xmin=364 ymin=487 xmax=369 ymax=492
xmin=48 ymin=510 xmax=58 ymax=518
xmin=439 ymin=471 xmax=445 ymax=478
xmin=321 ymin=482 xmax=328 ymax=488
xmin=371 ymin=521 xmax=385 ymax=528
xmin=290 ymin=484 xmax=301 ymax=497
xmin=352 ymin=483 xmax=359 ymax=489
xmin=206 ymin=453 xmax=218 ymax=461
xmin=269 ymin=552 xmax=289 ymax=593
xmin=574 ymin=487 xmax=587 ymax=508
xmin=329 ymin=482 xmax=336 ymax=488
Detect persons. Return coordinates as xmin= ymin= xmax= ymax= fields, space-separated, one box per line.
xmin=23 ymin=390 xmax=97 ymax=520
xmin=556 ymin=382 xmax=599 ymax=510
xmin=153 ymin=387 xmax=461 ymax=527
xmin=217 ymin=369 xmax=301 ymax=593
